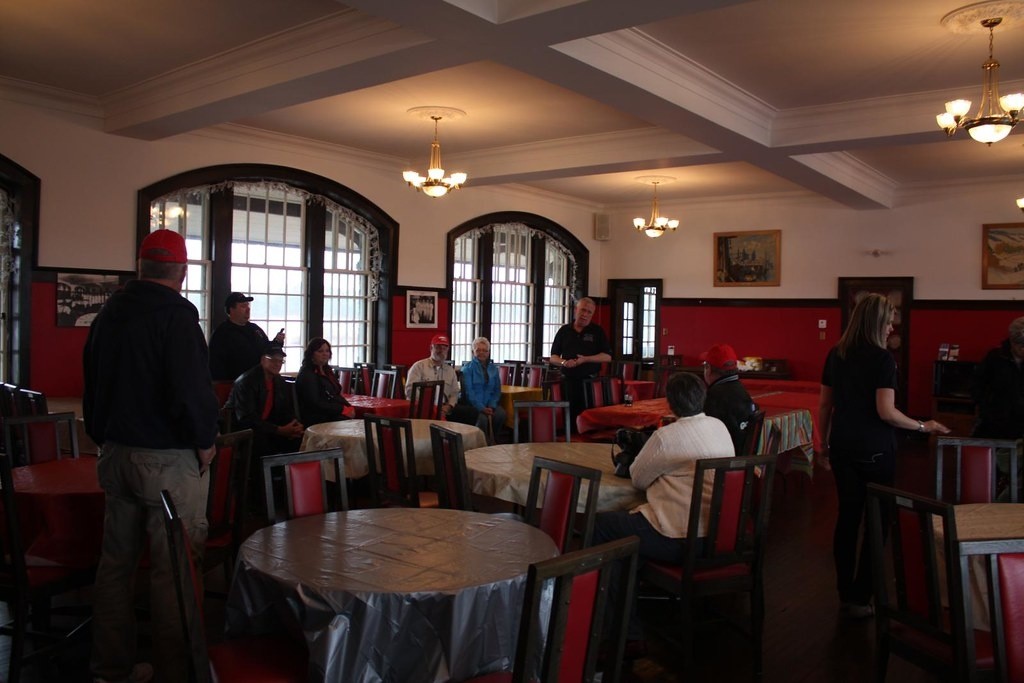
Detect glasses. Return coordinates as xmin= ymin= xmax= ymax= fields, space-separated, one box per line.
xmin=474 ymin=348 xmax=489 ymax=353
xmin=703 ymin=360 xmax=714 ymax=370
xmin=433 ymin=346 xmax=449 ymax=350
xmin=265 ymin=355 xmax=285 ymax=364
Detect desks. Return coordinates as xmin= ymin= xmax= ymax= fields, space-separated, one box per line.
xmin=464 ymin=442 xmax=647 ymax=518
xmin=339 ymin=393 xmax=448 ymax=423
xmin=217 ymin=507 xmax=562 ymax=683
xmin=298 ymin=418 xmax=488 ymax=510
xmin=0 ymin=455 xmax=107 ymax=589
xmin=930 ymin=503 xmax=1024 ymax=632
xmin=500 ymin=384 xmax=550 ymax=445
xmin=576 ymin=389 xmax=823 ymax=545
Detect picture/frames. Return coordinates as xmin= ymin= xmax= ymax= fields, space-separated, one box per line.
xmin=982 ymin=222 xmax=1024 ymax=290
xmin=712 ymin=229 xmax=782 ymax=289
xmin=405 ymin=289 xmax=438 ymax=329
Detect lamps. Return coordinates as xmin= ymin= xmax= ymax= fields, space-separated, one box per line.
xmin=935 ymin=0 xmax=1024 ymax=148
xmin=633 ymin=175 xmax=679 ymax=239
xmin=402 ymin=106 xmax=468 ymax=200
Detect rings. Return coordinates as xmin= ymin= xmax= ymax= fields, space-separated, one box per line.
xmin=209 ymin=461 xmax=211 ymax=464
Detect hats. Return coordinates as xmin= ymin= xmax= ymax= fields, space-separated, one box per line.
xmin=431 ymin=334 xmax=450 ymax=348
xmin=262 ymin=342 xmax=286 ymax=357
xmin=700 ymin=343 xmax=738 ymax=371
xmin=225 ymin=291 xmax=253 ymax=307
xmin=140 ymin=229 xmax=190 ymax=263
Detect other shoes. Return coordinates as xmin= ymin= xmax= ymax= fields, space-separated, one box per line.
xmin=92 ymin=663 xmax=153 ymax=683
xmin=846 ymin=601 xmax=876 ymax=618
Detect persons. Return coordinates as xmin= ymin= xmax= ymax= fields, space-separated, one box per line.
xmin=459 ymin=337 xmax=506 ymax=443
xmin=404 ymin=334 xmax=479 ymax=427
xmin=224 ymin=345 xmax=305 ymax=516
xmin=208 ymin=292 xmax=284 ymax=382
xmin=969 ymin=317 xmax=1024 ymax=498
xmin=550 ymin=297 xmax=612 ymax=436
xmin=293 ymin=338 xmax=355 ymax=428
xmin=83 ymin=229 xmax=219 ymax=683
xmin=411 ymin=299 xmax=433 ymax=323
xmin=697 ymin=343 xmax=759 ymax=455
xmin=579 ymin=370 xmax=736 ymax=660
xmin=819 ymin=293 xmax=952 ymax=618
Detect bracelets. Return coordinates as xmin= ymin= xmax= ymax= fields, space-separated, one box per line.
xmin=561 ymin=360 xmax=566 ymax=367
xmin=919 ymin=421 xmax=924 ymax=431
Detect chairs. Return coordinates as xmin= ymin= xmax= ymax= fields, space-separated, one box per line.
xmin=0 ymin=354 xmax=1024 ymax=683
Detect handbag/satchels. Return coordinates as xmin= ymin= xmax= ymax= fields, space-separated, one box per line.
xmin=610 ymin=424 xmax=659 ymax=479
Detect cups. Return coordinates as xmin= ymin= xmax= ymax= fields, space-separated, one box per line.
xmin=624 ymin=395 xmax=634 ymax=406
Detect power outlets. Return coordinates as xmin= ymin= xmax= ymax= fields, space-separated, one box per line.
xmin=663 ymin=328 xmax=668 ymax=336
xmin=820 ymin=332 xmax=825 ymax=340
